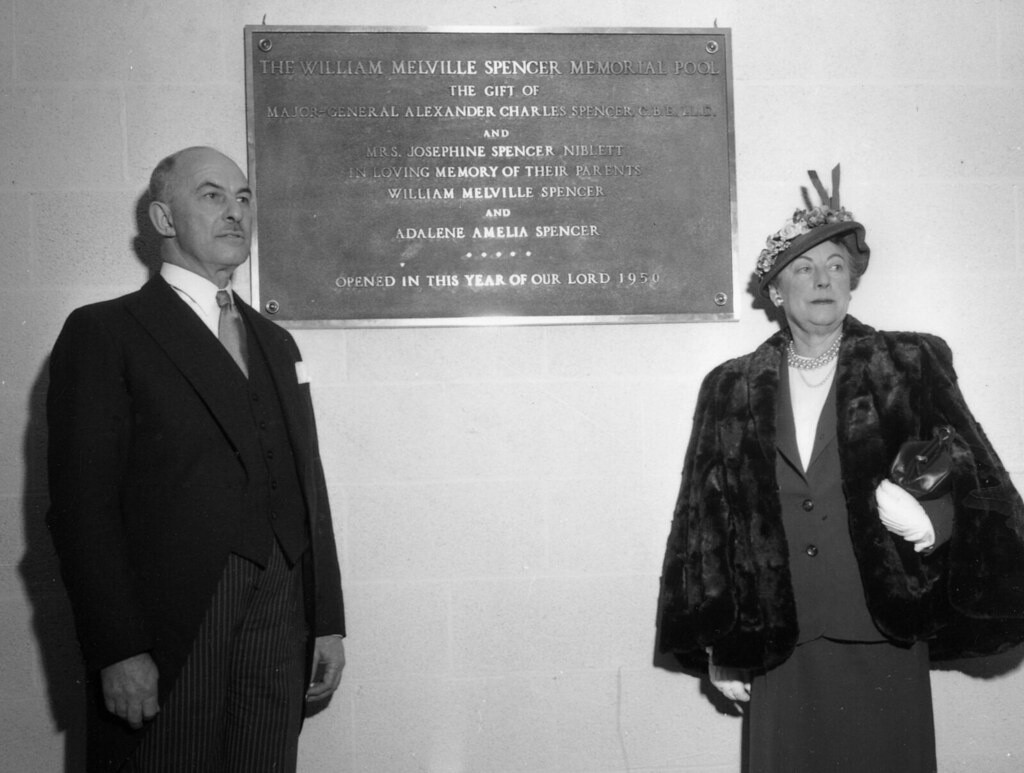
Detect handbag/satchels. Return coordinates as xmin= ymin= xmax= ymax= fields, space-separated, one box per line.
xmin=889 ymin=423 xmax=994 ymax=608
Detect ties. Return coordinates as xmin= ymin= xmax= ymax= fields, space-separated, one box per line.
xmin=215 ymin=291 xmax=250 ymax=382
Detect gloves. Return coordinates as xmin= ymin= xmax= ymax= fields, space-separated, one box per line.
xmin=705 ymin=645 xmax=751 ymax=702
xmin=875 ymin=478 xmax=935 ymax=553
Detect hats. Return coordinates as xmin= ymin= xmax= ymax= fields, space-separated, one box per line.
xmin=752 ymin=162 xmax=870 ymax=298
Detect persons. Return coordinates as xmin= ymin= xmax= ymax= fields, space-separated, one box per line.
xmin=652 ymin=165 xmax=1024 ymax=773
xmin=44 ymin=145 xmax=347 ymax=773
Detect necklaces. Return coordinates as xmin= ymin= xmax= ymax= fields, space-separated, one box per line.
xmin=786 ymin=336 xmax=841 ymax=389
